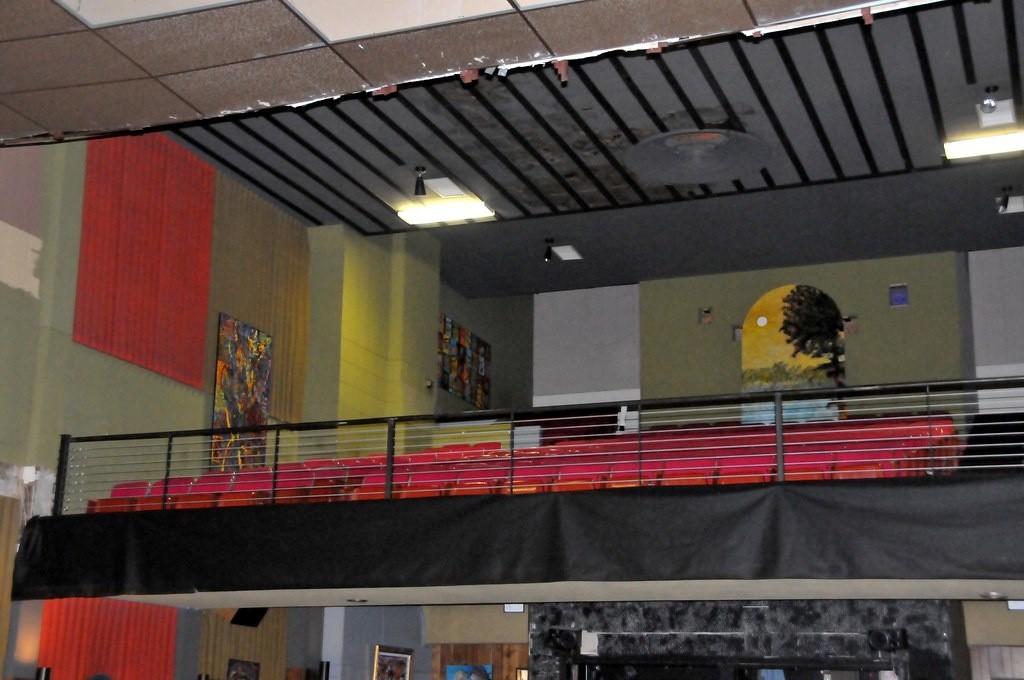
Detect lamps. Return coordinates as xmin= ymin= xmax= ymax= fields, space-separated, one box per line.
xmin=35 ymin=666 xmax=52 ymax=680
xmin=397 ymin=201 xmax=495 ymax=225
xmin=943 ymin=127 xmax=1024 ymax=159
xmin=545 ymin=628 xmax=583 ymax=654
xmin=319 ymin=661 xmax=331 ymax=680
xmin=543 ymin=237 xmax=554 ymax=262
xmin=981 ymin=86 xmax=1000 ymax=113
xmin=414 ymin=166 xmax=427 ymax=196
xmin=867 ymin=627 xmax=908 ymax=651
xmin=996 ymin=184 xmax=1013 ymax=213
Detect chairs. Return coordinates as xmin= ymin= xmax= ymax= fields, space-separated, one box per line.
xmin=82 ymin=411 xmax=961 ymax=511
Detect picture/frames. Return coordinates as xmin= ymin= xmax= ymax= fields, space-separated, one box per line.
xmin=372 ymin=645 xmax=415 ymax=680
xmin=226 ymin=658 xmax=260 ymax=680
